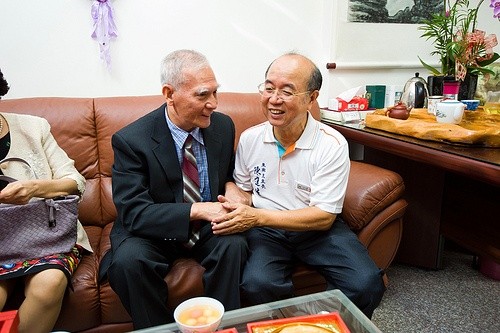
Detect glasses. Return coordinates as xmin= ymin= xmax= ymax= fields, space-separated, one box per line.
xmin=256 ymin=82 xmax=312 ymax=101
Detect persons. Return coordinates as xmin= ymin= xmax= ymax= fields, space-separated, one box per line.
xmin=212 ymin=52 xmax=385 ymax=323
xmin=0 ymin=68 xmax=94 ymax=333
xmin=98 ymin=49 xmax=250 ymax=331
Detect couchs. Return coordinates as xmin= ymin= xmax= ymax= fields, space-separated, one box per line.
xmin=0 ymin=92 xmax=409 ymax=333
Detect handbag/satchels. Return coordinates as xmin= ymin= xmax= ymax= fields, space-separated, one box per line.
xmin=1 ymin=158 xmax=80 ymax=263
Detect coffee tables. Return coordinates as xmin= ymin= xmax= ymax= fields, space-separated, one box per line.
xmin=130 ymin=289 xmax=382 ymax=333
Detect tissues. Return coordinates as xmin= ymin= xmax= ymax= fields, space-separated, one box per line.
xmin=328 ymin=86 xmax=368 ymax=112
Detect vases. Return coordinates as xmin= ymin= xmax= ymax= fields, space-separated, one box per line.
xmin=427 ymin=75 xmax=478 ymax=102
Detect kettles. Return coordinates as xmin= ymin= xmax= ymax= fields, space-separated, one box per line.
xmin=400 ymin=72 xmax=430 ymax=110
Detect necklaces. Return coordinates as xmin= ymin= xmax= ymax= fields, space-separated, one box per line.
xmin=0 ymin=116 xmax=3 ymax=135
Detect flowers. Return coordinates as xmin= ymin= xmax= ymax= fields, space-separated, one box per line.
xmin=417 ymin=0 xmax=500 ymax=82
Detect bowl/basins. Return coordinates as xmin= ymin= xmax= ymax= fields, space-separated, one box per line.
xmin=462 ymin=99 xmax=479 ymax=111
xmin=174 ymin=297 xmax=225 ymax=333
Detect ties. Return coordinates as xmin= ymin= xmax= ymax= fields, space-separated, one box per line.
xmin=182 ymin=134 xmax=201 ymax=250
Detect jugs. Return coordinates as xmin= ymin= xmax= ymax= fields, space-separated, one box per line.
xmin=484 ymin=91 xmax=500 ymax=116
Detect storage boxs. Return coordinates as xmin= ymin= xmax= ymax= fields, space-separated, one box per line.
xmin=366 ymin=85 xmax=386 ymax=109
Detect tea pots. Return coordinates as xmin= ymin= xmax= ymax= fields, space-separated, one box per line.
xmin=434 ymin=98 xmax=467 ymax=125
xmin=386 ymin=102 xmax=413 ymax=120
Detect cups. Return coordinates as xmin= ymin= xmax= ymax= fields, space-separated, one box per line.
xmin=429 ymin=96 xmax=443 ymax=113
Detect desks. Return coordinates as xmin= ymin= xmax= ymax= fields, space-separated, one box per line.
xmin=320 ymin=117 xmax=500 ymax=272
